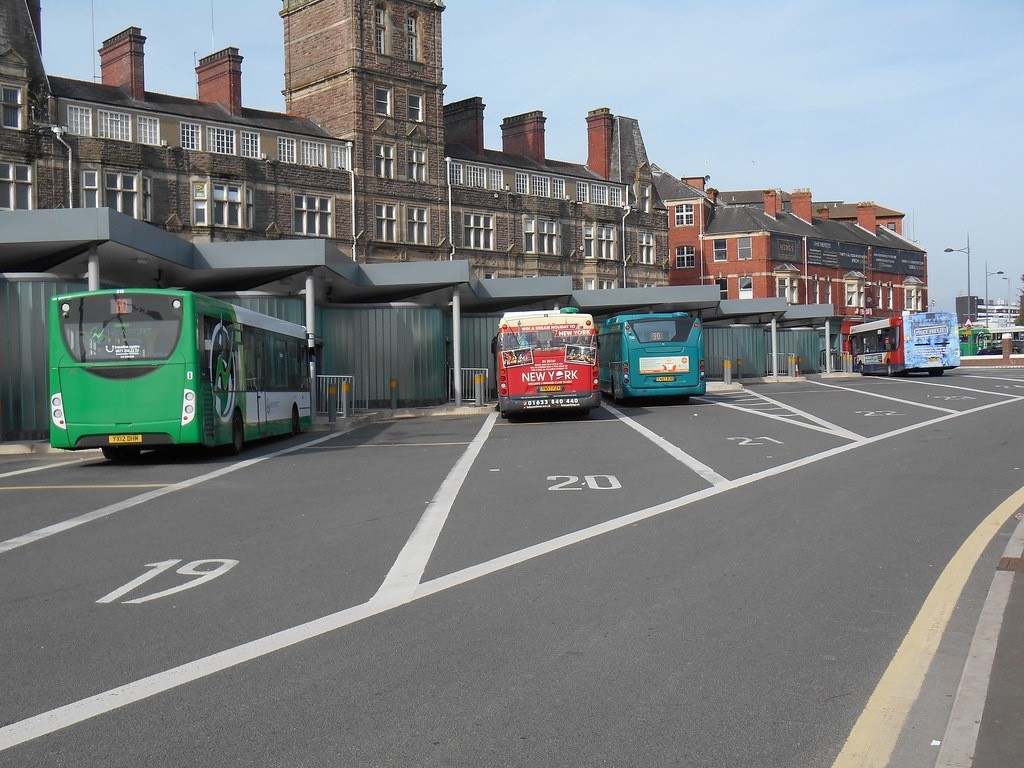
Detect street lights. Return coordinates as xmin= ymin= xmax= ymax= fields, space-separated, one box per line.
xmin=943 ymin=232 xmax=970 ymax=321
xmin=1003 ymin=277 xmax=1011 ymax=326
xmin=985 ymin=260 xmax=1005 ymax=327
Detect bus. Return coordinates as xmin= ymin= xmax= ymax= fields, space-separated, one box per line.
xmin=958 ymin=325 xmax=1024 ymax=356
xmin=47 ymin=288 xmax=318 ymax=461
xmin=591 ymin=312 xmax=706 ymax=406
xmin=847 ymin=311 xmax=961 ymax=378
xmin=490 ymin=305 xmax=601 ymax=419
